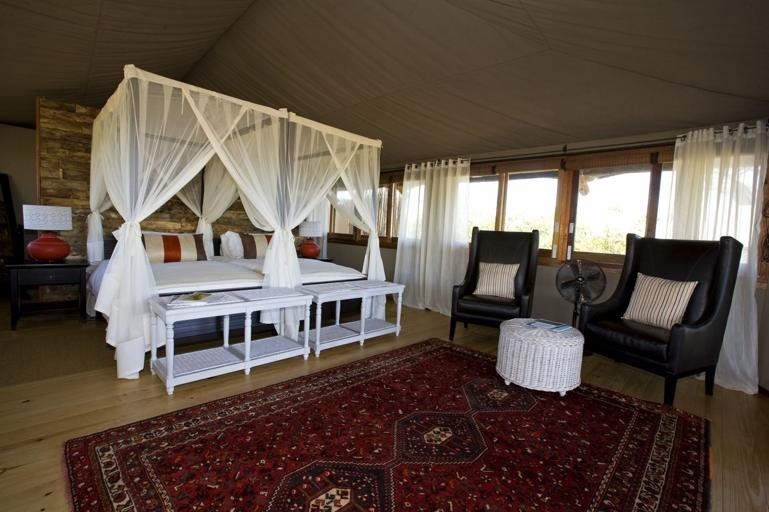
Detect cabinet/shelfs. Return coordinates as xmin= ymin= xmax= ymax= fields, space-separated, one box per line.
xmin=147 ymin=280 xmax=407 ymax=395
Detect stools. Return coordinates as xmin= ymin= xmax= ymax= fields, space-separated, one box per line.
xmin=495 ymin=318 xmax=585 ymax=397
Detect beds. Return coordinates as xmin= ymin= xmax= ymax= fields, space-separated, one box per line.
xmin=88 ymin=237 xmax=381 ymax=349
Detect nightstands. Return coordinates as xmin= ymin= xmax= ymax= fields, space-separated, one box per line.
xmin=5 ymin=260 xmax=90 ymax=331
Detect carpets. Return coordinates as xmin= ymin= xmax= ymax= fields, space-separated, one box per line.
xmin=60 ymin=337 xmax=713 ymax=512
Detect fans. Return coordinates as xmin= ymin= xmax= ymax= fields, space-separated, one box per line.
xmin=555 ymin=259 xmax=607 ymax=357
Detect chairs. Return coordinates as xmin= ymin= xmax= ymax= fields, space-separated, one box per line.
xmin=578 ymin=233 xmax=744 ymax=406
xmin=449 ymin=227 xmax=539 ymax=341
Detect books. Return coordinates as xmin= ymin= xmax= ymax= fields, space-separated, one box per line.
xmin=167 ymin=291 xmax=214 ymax=306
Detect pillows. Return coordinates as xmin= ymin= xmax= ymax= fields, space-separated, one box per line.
xmin=219 ymin=231 xmax=272 ymax=259
xmin=620 ymin=272 xmax=699 ymax=332
xmin=472 ymin=262 xmax=520 ymax=300
xmin=142 ymin=234 xmax=208 ymax=262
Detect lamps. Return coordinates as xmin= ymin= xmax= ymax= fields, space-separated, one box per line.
xmin=22 ymin=204 xmax=72 ymax=261
